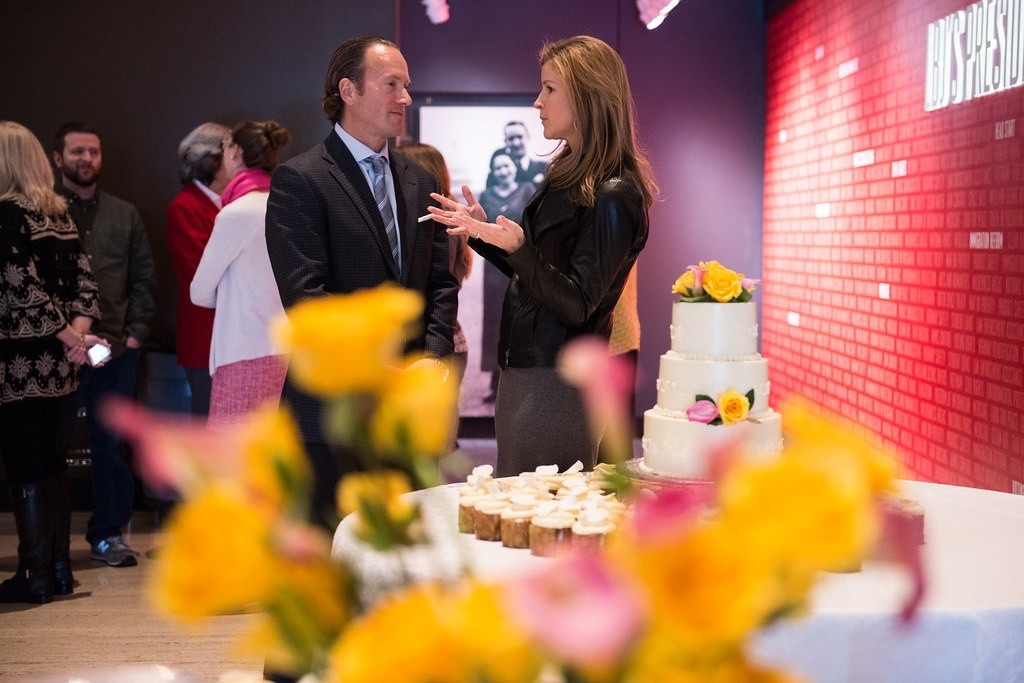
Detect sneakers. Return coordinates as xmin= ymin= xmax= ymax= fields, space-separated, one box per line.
xmin=89 ymin=534 xmax=139 ymax=568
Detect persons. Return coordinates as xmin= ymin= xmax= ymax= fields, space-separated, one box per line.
xmin=49 ymin=122 xmax=159 ymax=568
xmin=427 ymin=35 xmax=663 ymax=480
xmin=165 ymin=121 xmax=232 ymax=414
xmin=264 ymin=37 xmax=461 ymax=539
xmin=478 ymin=154 xmax=538 ymax=404
xmin=392 ymin=143 xmax=473 ymax=385
xmin=189 ymin=119 xmax=292 ymax=429
xmin=0 ymin=121 xmax=112 ymax=603
xmin=486 ymin=122 xmax=551 ymax=190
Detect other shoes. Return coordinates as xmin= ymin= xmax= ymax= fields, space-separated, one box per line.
xmin=0 ymin=567 xmax=55 ymax=605
xmin=52 ymin=567 xmax=74 ymax=596
xmin=481 ymin=388 xmax=496 ymax=403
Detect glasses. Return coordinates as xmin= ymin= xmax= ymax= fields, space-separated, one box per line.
xmin=221 ymin=139 xmax=233 ymax=150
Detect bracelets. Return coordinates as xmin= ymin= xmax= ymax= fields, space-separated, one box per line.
xmin=76 ymin=332 xmax=86 ymax=348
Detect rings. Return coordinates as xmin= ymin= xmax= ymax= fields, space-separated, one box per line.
xmin=475 ymin=233 xmax=480 ymax=239
xmin=67 ymin=358 xmax=71 ymax=362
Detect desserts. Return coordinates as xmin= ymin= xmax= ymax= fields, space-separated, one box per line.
xmin=457 ymin=462 xmax=631 ymax=558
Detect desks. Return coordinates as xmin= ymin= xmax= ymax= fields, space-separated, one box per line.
xmin=319 ymin=476 xmax=1023 ymax=683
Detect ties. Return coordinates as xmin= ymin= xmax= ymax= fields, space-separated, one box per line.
xmin=366 ymin=154 xmax=401 ymax=266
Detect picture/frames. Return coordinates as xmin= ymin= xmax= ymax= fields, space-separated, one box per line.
xmin=386 ymin=92 xmax=609 ymax=443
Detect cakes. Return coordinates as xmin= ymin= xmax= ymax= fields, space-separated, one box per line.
xmin=642 ymin=258 xmax=782 ymax=481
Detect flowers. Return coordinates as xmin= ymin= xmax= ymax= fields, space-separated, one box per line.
xmin=686 ymin=389 xmax=758 ymax=425
xmin=669 ymin=259 xmax=764 ymax=303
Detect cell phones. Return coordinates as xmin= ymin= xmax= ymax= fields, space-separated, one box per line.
xmin=87 ymin=338 xmax=111 ymax=367
